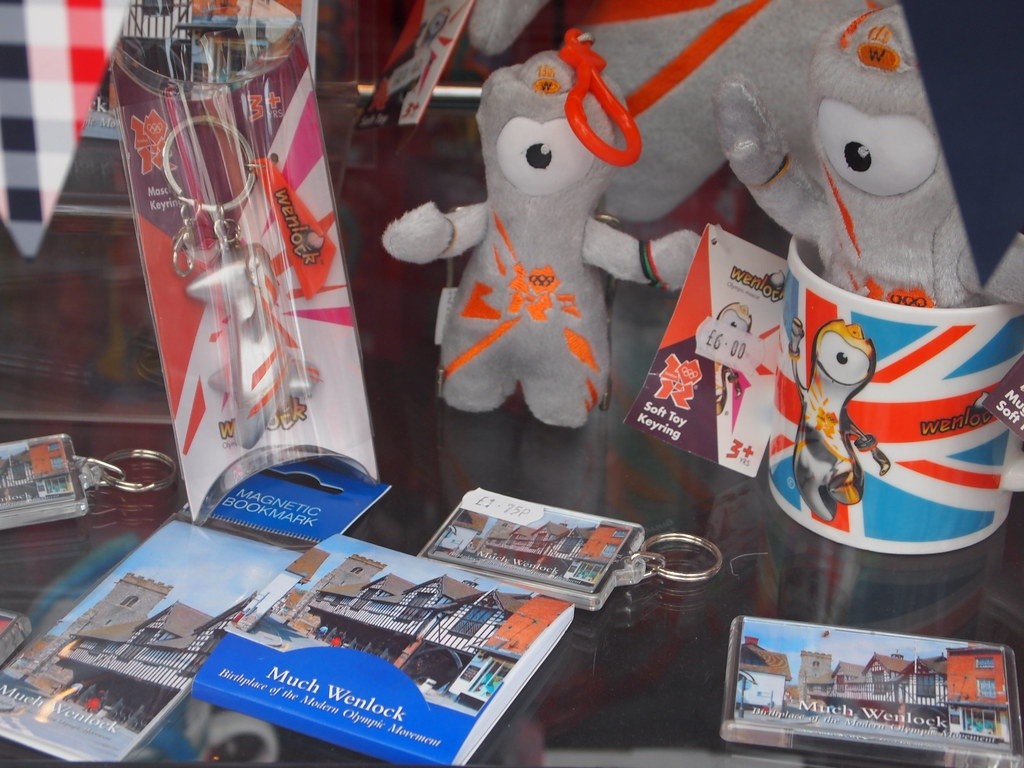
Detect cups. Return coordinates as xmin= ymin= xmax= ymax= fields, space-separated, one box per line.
xmin=769 ymin=232 xmax=1024 ymax=554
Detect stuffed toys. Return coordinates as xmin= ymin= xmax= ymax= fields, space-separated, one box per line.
xmin=466 ymin=0 xmax=901 ymax=222
xmin=381 ymin=50 xmax=701 ymax=427
xmin=715 ymin=6 xmax=1024 ymax=308
